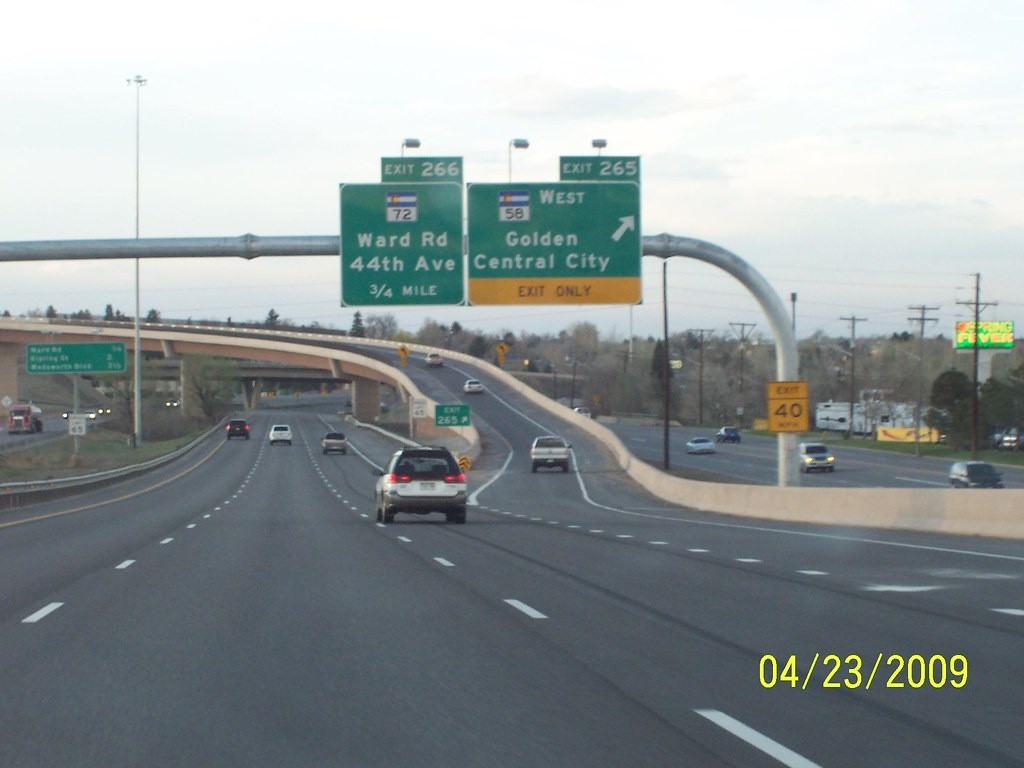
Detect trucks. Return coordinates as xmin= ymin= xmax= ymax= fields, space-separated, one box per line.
xmin=5 ymin=404 xmax=44 ymax=436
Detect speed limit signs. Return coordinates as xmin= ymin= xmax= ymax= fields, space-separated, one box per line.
xmin=766 ymin=379 xmax=810 ymax=434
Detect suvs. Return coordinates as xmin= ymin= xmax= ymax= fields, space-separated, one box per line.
xmin=529 ymin=435 xmax=574 ymax=474
xmin=371 ymin=445 xmax=469 ymax=524
xmin=268 ymin=424 xmax=293 ymax=447
xmin=798 ymin=442 xmax=836 ymax=474
xmin=425 ymin=352 xmax=445 ymax=367
xmin=946 ymin=459 xmax=1004 ymax=489
xmin=224 ymin=418 xmax=250 ymax=440
xmin=320 ymin=432 xmax=348 ymax=456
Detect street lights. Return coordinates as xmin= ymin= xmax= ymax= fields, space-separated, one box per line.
xmin=126 ymin=73 xmax=151 ymax=448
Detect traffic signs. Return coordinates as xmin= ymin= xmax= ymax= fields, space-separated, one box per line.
xmin=434 ymin=404 xmax=472 ymax=427
xmin=463 ymin=153 xmax=647 ymax=307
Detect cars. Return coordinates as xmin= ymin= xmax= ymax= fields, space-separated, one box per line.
xmin=61 ymin=405 xmax=112 ymax=420
xmin=164 ymin=399 xmax=181 ymax=408
xmin=715 ymin=427 xmax=742 ymax=445
xmin=686 ymin=438 xmax=716 ymax=455
xmin=573 ymin=407 xmax=592 ymax=418
xmin=463 ymin=380 xmax=485 ymax=394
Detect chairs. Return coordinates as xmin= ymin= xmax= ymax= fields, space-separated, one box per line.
xmin=399 ymin=464 xmax=418 ymax=474
xmin=431 ymin=464 xmax=448 ymax=474
